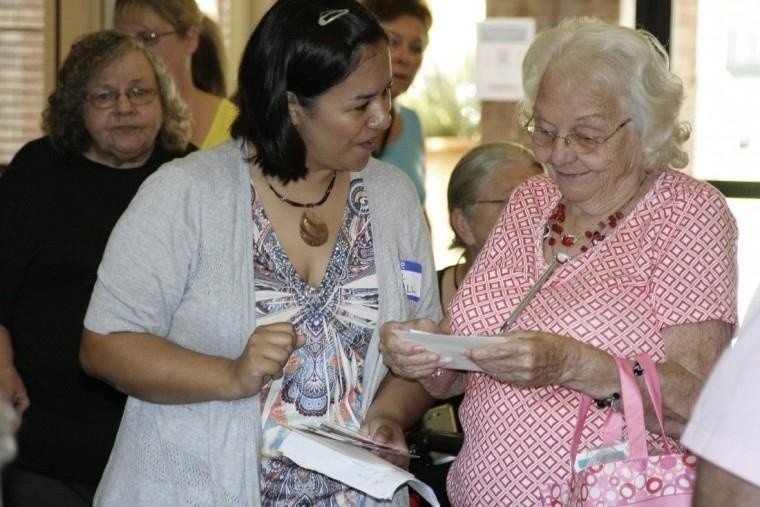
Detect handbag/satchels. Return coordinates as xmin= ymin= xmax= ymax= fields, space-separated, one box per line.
xmin=568 ymin=356 xmax=698 ymax=506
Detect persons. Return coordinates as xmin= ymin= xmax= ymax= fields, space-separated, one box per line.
xmin=75 ymin=0 xmax=444 ymax=507
xmin=378 ymin=14 xmax=737 ymax=507
xmin=398 ymin=143 xmax=542 ymax=506
xmin=676 ymin=283 xmax=760 ymax=507
xmin=1 ymin=323 xmax=31 ymax=415
xmin=0 ymin=29 xmax=203 ymax=507
xmin=107 ymin=1 xmax=239 ymax=148
xmin=369 ymin=0 xmax=431 ymax=242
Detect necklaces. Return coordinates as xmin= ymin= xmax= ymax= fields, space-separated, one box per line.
xmin=544 ymin=172 xmax=657 ymax=264
xmin=258 ymin=163 xmax=334 ymax=247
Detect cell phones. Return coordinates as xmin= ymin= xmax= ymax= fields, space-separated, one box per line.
xmin=424 ymin=404 xmax=460 ymax=459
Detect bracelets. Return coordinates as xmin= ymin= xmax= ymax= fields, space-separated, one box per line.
xmin=591 ymin=358 xmax=644 ymax=413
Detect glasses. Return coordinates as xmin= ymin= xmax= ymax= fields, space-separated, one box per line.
xmin=522 ymin=115 xmax=633 ymax=155
xmin=82 ymin=87 xmax=160 ymax=109
xmin=136 ymin=30 xmax=180 ymax=46
